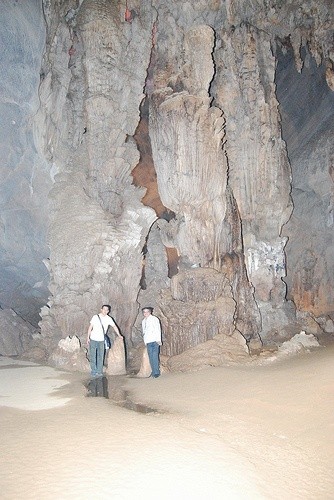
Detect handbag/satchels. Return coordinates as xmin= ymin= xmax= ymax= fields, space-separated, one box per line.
xmin=105 ymin=334 xmax=111 ymax=350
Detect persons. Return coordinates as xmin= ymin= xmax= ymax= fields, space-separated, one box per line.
xmin=141 ymin=307 xmax=163 ymax=379
xmin=87 ymin=304 xmax=123 ymax=377
